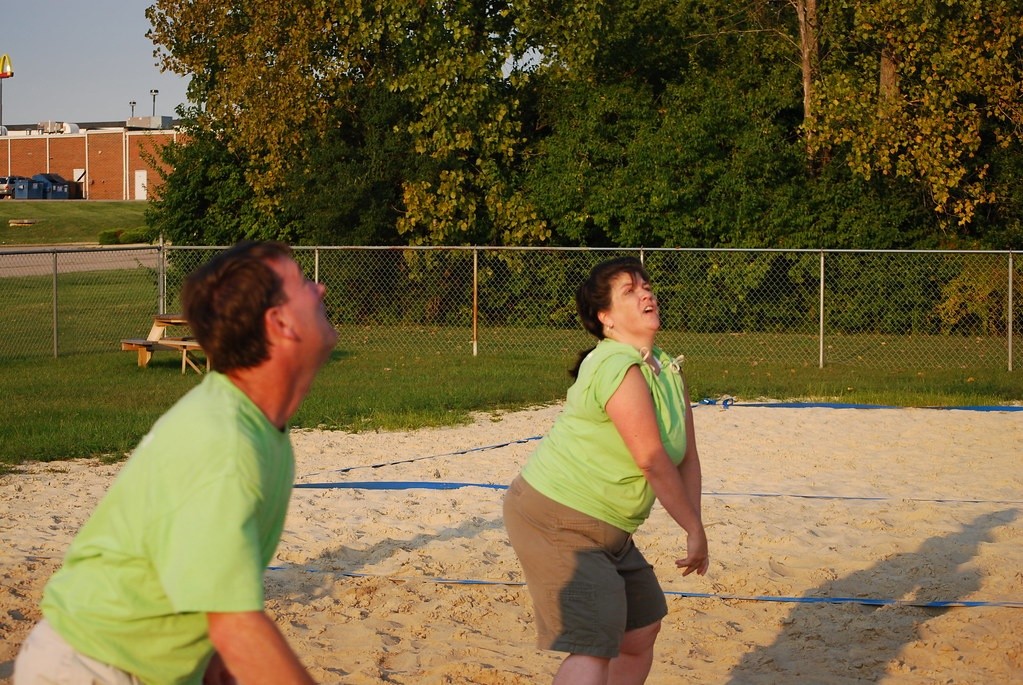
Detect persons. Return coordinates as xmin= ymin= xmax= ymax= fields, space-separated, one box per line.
xmin=12 ymin=240 xmax=336 ymax=685
xmin=503 ymin=256 xmax=709 ymax=685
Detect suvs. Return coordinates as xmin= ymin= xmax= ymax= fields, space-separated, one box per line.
xmin=0 ymin=175 xmax=32 ymax=200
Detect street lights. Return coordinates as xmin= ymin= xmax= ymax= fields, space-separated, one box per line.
xmin=149 ymin=89 xmax=159 ymax=115
xmin=129 ymin=101 xmax=137 ymax=117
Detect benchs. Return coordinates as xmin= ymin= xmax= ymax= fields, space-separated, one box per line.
xmin=120 ymin=314 xmax=212 ymax=376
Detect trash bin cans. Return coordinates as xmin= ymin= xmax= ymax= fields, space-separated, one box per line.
xmin=32 ymin=173 xmax=81 ymax=199
xmin=13 ymin=179 xmax=43 ymax=199
